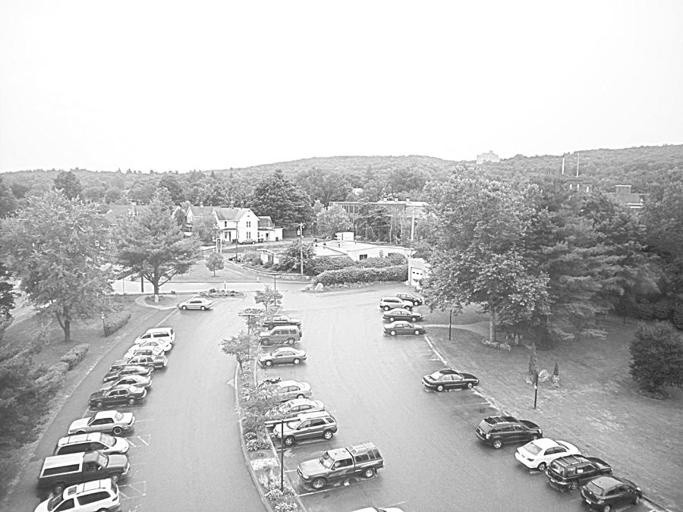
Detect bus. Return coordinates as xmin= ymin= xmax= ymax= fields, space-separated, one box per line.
xmin=108 ymin=265 xmax=131 ymax=279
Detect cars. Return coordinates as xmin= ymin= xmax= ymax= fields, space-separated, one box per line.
xmin=33 ymin=327 xmax=175 ymax=512
xmin=239 ymin=239 xmax=255 ymax=244
xmin=420 ymin=367 xmax=478 ymax=392
xmin=350 ymin=506 xmax=403 ymax=512
xmin=257 ymin=311 xmax=308 ymax=365
xmin=380 ymin=294 xmax=426 ymax=339
xmin=471 ymin=414 xmax=645 ymax=512
xmin=261 ymin=379 xmax=336 ymax=443
xmin=177 ymin=299 xmax=212 ymax=311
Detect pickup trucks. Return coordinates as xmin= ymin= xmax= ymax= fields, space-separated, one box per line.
xmin=293 ymin=440 xmax=386 ymax=489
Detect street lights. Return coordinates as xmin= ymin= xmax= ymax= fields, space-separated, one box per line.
xmin=99 ymin=310 xmax=108 ymax=337
xmin=443 ymin=295 xmax=460 ymax=341
xmin=296 ymin=222 xmax=307 ymax=277
